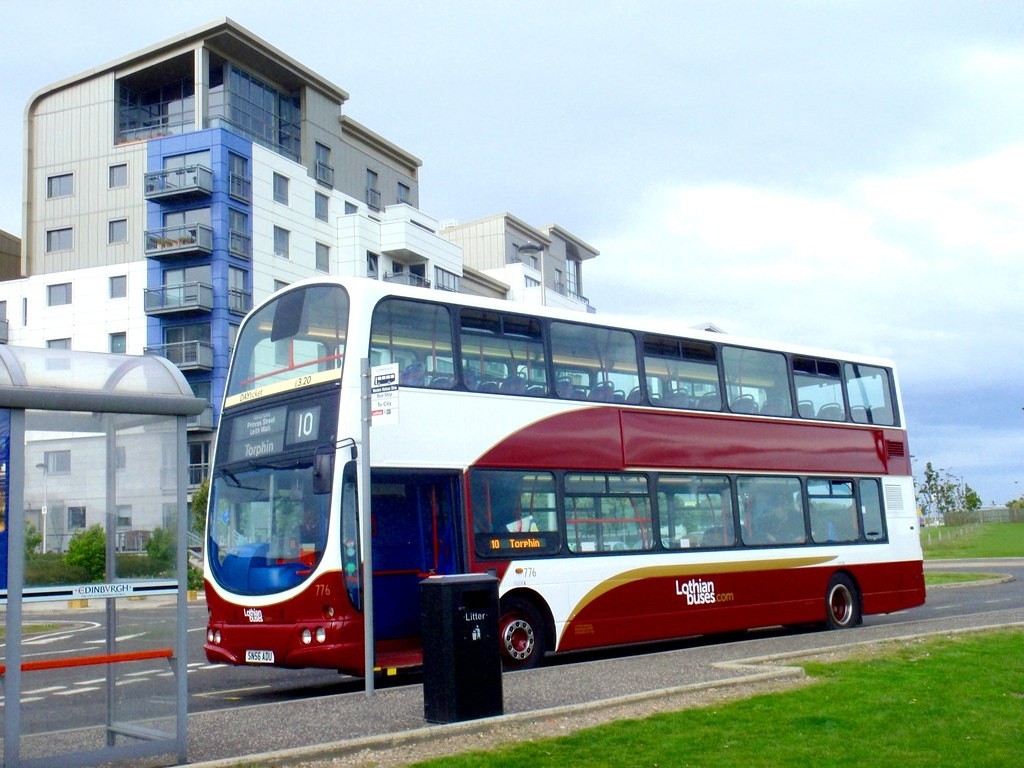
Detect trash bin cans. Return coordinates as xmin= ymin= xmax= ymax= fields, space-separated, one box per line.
xmin=420 ymin=572 xmax=505 ymax=726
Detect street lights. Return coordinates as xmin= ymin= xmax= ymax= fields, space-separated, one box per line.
xmin=35 ymin=463 xmax=49 ymax=555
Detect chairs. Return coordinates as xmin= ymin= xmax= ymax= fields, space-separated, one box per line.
xmin=398 ymin=360 xmax=891 ymax=427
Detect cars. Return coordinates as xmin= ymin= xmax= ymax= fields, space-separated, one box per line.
xmin=566 ymin=530 xmax=704 ymax=552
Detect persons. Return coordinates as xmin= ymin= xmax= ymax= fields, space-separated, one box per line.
xmin=287 ymin=509 xmax=321 ymax=547
xmin=758 ymin=503 xmax=796 ymax=545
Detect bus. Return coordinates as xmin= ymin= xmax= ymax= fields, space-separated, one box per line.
xmin=200 ymin=275 xmax=928 ymax=679
